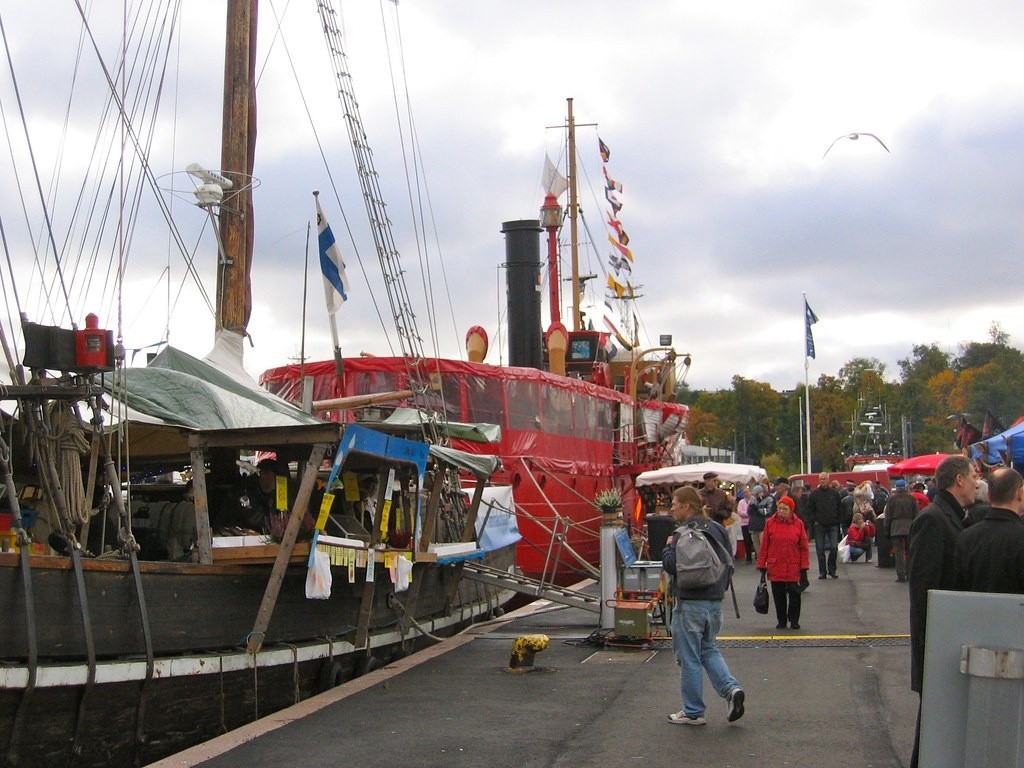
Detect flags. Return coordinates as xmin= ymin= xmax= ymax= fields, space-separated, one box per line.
xmin=805 ymin=299 xmax=821 ymax=360
xmin=955 ymin=409 xmax=1006 ymax=449
xmin=315 ymin=197 xmax=348 ymax=317
xmin=595 ymin=138 xmax=637 ymax=303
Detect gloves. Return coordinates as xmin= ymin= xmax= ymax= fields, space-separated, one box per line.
xmin=759 ymin=568 xmax=767 ymax=574
xmin=795 ymin=571 xmax=809 ymax=592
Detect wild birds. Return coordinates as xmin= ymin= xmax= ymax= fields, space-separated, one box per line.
xmin=822 ymin=133 xmax=891 ymax=159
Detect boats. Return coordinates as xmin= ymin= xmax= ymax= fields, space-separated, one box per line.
xmin=258 ymin=77 xmax=695 ymax=600
xmin=2 ymin=0 xmax=525 ymax=767
xmin=840 ymin=367 xmax=909 ymax=472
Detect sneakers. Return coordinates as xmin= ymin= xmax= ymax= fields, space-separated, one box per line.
xmin=667 ymin=709 xmax=706 ymax=726
xmin=776 ymin=622 xmax=787 ymax=629
xmin=790 ymin=622 xmax=801 ymax=630
xmin=726 ymin=688 xmax=746 ymax=722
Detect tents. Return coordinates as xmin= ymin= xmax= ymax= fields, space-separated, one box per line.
xmin=965 ymin=420 xmax=1024 ymax=469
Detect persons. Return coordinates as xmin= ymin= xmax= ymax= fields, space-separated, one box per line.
xmin=697 ymin=468 xmax=990 ymax=554
xmin=660 ymin=485 xmax=747 ymax=728
xmin=171 ymin=479 xmax=196 ymax=502
xmin=808 ymin=471 xmax=844 ymax=579
xmin=73 ymin=483 xmax=122 ymax=558
xmin=844 ymin=512 xmax=877 ymax=564
xmin=883 ymin=477 xmax=919 ymax=583
xmin=906 ymin=455 xmax=981 ymax=767
xmin=754 ymin=496 xmax=811 ymax=631
xmin=953 ymin=463 xmax=1024 ymax=596
xmin=222 ymin=457 xmax=325 ymax=540
xmin=327 ymin=472 xmax=381 ymax=532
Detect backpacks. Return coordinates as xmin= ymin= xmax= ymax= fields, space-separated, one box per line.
xmin=673 ymin=526 xmax=734 ymax=592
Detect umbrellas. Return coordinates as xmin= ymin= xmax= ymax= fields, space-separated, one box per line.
xmin=633 ymin=458 xmax=765 ymax=492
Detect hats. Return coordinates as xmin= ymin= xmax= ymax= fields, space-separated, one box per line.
xmin=847 ymin=479 xmax=855 ymax=483
xmin=774 ymin=476 xmax=788 ymax=485
xmin=895 ymin=480 xmax=906 ymax=487
xmin=778 ymin=496 xmax=795 ymax=513
xmin=924 ymin=478 xmax=931 ymax=484
xmin=360 ymin=474 xmax=378 ymax=485
xmin=703 ymin=473 xmax=718 ymax=479
xmin=752 ymin=485 xmax=763 ymax=495
xmin=256 ymin=458 xmax=285 ymax=476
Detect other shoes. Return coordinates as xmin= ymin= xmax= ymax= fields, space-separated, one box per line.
xmin=818 ymin=572 xmax=826 ymax=578
xmin=828 ymin=570 xmax=838 ymax=578
xmin=865 ymin=559 xmax=874 ymax=563
xmin=894 ymin=577 xmax=906 ymax=582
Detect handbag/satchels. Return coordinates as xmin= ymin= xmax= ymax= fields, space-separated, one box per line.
xmin=838 ymin=533 xmax=852 ymax=563
xmin=753 ymin=573 xmax=770 ymax=615
